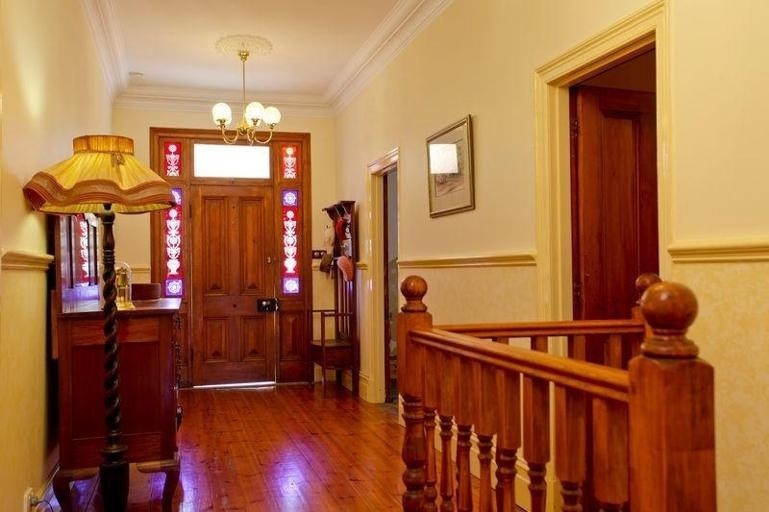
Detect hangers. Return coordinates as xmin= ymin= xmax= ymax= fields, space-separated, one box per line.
xmin=322 ymin=200 xmax=356 ymax=264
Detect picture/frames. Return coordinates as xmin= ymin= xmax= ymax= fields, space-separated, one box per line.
xmin=425 ymin=113 xmax=475 ymax=218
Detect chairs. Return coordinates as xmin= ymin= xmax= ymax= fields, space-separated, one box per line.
xmin=307 ymin=256 xmax=360 ymax=399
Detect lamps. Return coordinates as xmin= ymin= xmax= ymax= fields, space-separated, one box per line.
xmin=23 ymin=134 xmax=176 ymax=512
xmin=211 ymin=35 xmax=281 ymax=145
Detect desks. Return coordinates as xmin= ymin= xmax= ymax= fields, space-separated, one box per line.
xmin=49 ymin=298 xmax=184 ymax=512
